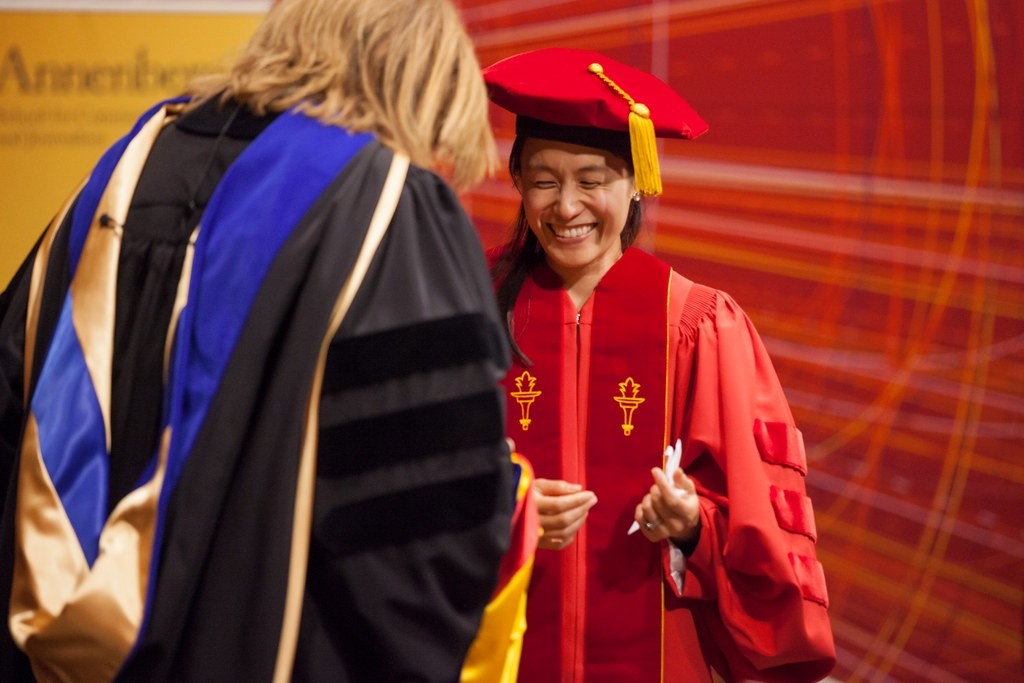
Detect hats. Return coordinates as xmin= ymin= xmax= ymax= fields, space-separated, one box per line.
xmin=482 ymin=49 xmax=711 ymax=197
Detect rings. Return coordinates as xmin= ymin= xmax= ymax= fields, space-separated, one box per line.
xmin=645 ymin=520 xmax=663 ymax=530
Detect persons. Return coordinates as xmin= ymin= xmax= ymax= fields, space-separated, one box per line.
xmin=488 ymin=43 xmax=840 ymax=683
xmin=0 ymin=0 xmax=543 ymax=683
